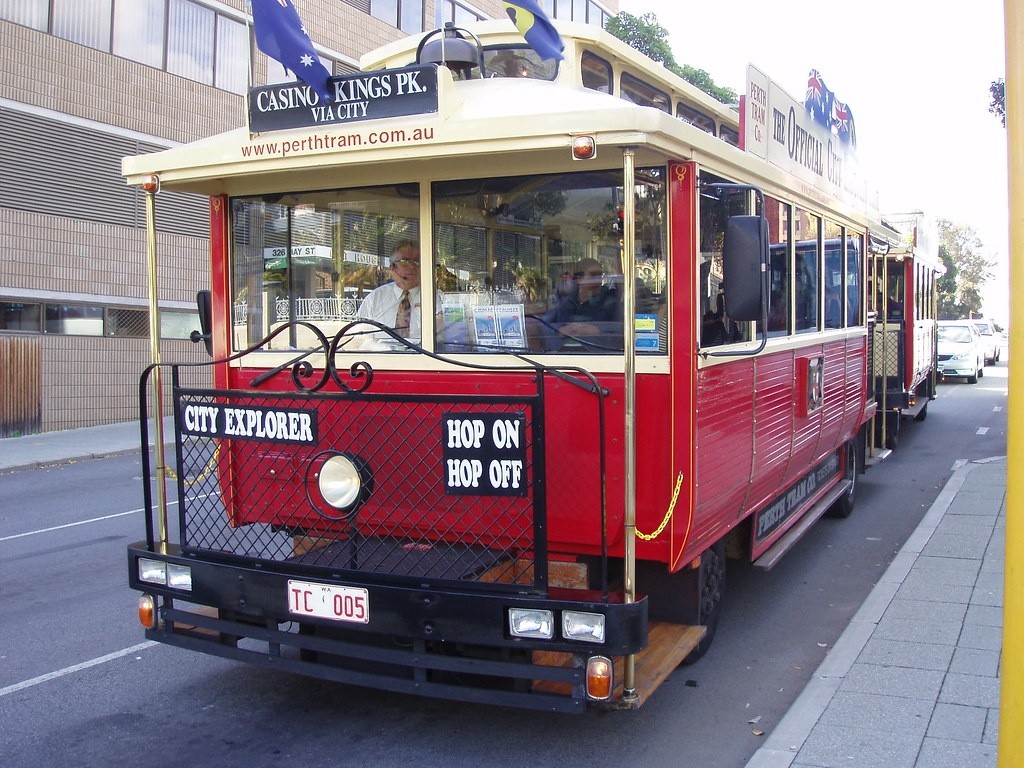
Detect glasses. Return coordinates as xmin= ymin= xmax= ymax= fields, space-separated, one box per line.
xmin=394 ymin=257 xmax=419 ymax=266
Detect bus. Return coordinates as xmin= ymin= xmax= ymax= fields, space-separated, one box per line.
xmin=123 ymin=0 xmax=948 ymax=716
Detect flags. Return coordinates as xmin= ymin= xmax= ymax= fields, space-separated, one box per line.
xmin=502 ymin=0 xmax=566 ymax=62
xmin=251 ymin=0 xmax=331 ymax=105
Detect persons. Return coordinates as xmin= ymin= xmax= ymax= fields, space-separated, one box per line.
xmin=538 ymin=222 xmax=743 ymax=354
xmin=350 ymin=238 xmax=444 ymax=344
xmin=765 ymin=269 xmax=892 ymax=332
xmin=700 ymin=295 xmax=733 ymax=346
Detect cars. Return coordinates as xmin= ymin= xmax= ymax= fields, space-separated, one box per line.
xmin=956 ymin=319 xmax=1002 ymax=365
xmin=937 ymin=320 xmax=985 ymax=383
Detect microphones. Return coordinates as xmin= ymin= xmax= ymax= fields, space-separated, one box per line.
xmin=392 ymin=263 xmax=407 ymax=279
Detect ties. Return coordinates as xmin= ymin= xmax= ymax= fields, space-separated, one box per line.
xmin=396 ymin=291 xmax=411 ymax=337
